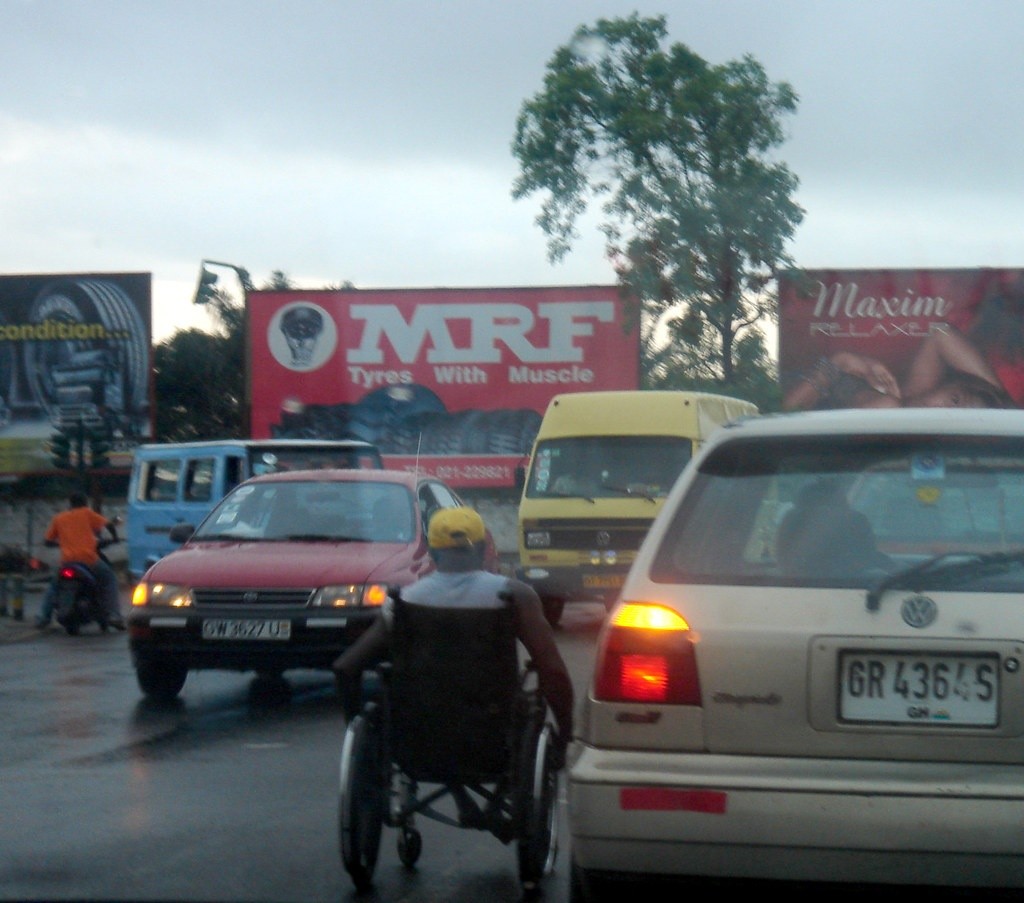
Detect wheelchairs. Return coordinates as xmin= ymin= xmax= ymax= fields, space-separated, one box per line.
xmin=339 ymin=589 xmax=562 ymax=901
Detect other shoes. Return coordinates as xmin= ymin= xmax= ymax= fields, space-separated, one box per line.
xmin=459 ymin=804 xmax=504 ymax=828
xmin=36 ymin=618 xmax=50 ymax=629
xmin=110 ymin=617 xmax=127 ymax=631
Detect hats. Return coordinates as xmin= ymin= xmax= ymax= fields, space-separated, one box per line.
xmin=427 ymin=504 xmax=484 ymax=547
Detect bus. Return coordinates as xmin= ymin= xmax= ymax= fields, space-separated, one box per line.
xmin=514 ymin=391 xmax=764 ymax=627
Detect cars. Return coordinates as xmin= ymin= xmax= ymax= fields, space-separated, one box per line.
xmin=125 ymin=471 xmax=499 ymax=701
xmin=555 ymin=407 xmax=1024 ymax=902
xmin=821 ymin=457 xmax=1022 ymax=583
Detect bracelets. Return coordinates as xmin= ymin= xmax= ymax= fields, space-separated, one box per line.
xmin=798 ymin=374 xmax=823 ymax=399
xmin=816 ymin=358 xmax=841 ymax=384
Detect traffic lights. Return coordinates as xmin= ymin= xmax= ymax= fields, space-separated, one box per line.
xmin=90 ymin=427 xmax=110 ymax=470
xmin=49 ymin=433 xmax=72 ymax=470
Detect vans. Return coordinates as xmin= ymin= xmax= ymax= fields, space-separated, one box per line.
xmin=126 ymin=439 xmax=381 ymax=581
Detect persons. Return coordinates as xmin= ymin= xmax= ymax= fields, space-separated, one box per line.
xmin=781 ymin=322 xmax=1016 ymax=411
xmin=34 ymin=492 xmax=125 ymax=631
xmin=335 ymin=508 xmax=574 ymax=826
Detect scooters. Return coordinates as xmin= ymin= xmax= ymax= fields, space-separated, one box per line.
xmin=56 ymin=540 xmax=114 ymax=636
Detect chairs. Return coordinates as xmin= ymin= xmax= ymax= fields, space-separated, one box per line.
xmin=779 ymin=504 xmax=881 ymax=585
